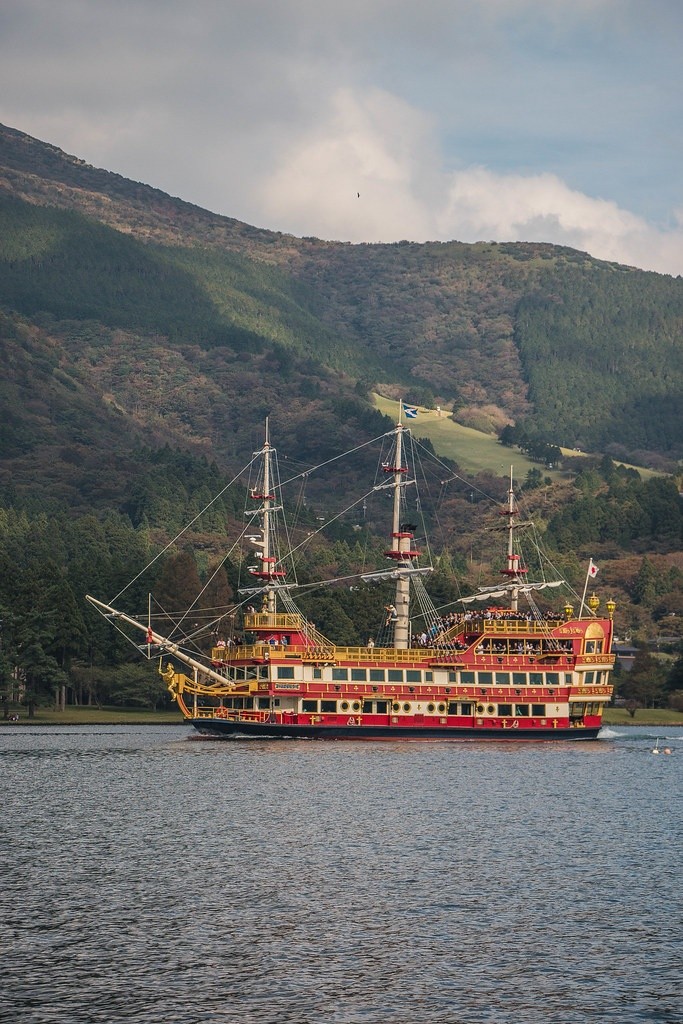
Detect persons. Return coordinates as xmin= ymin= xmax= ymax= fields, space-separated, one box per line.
xmin=217 ymin=636 xmax=242 ymax=647
xmin=412 ymin=609 xmax=573 ymax=654
xmin=516 ymin=706 xmax=522 ymax=716
xmin=268 ymin=634 xmax=277 ymax=644
xmin=244 ymin=605 xmax=268 ymax=625
xmin=281 ymin=635 xmax=288 ymax=647
xmin=385 ymin=606 xmax=398 ymax=626
xmin=368 ymin=638 xmax=374 ymax=648
xmin=587 ymin=642 xmax=599 ymax=653
xmin=9 ymin=713 xmax=19 ymax=721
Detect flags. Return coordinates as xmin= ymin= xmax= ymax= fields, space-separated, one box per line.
xmin=403 ymin=405 xmax=418 ymax=418
xmin=590 ymin=563 xmax=599 ymax=578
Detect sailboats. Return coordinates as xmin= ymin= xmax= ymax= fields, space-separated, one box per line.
xmin=84 ymin=397 xmax=619 ymax=742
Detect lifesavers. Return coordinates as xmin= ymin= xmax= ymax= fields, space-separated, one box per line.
xmin=216 ymin=706 xmax=228 ymax=718
